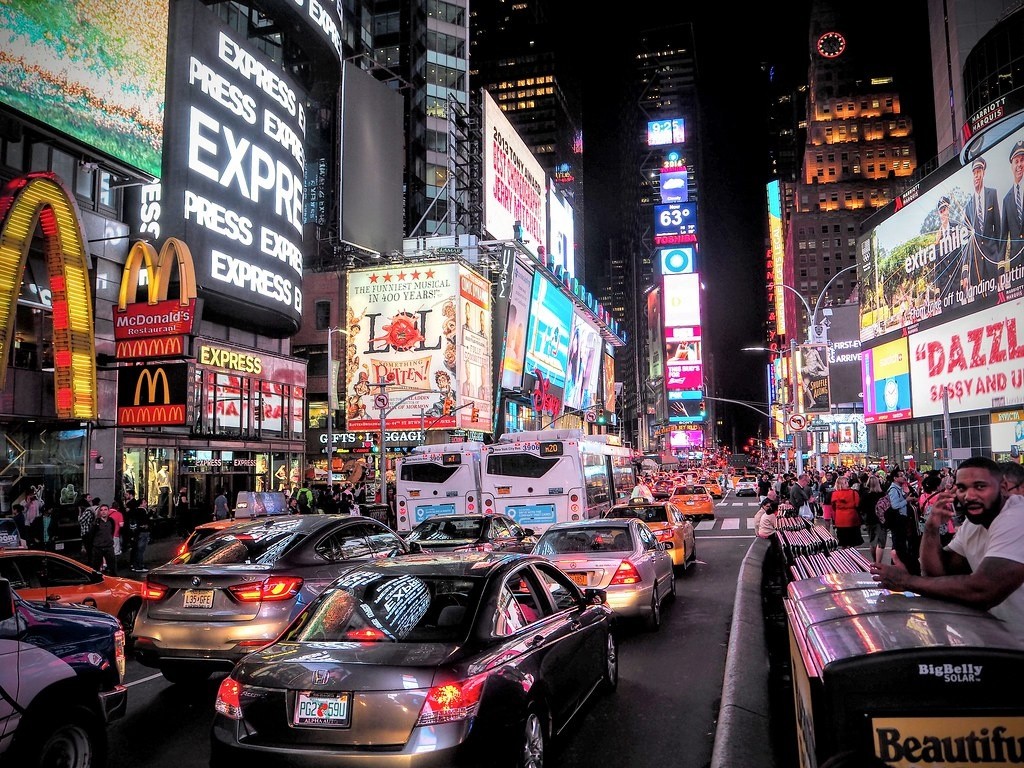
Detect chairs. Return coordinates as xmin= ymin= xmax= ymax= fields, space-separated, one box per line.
xmin=443 ymin=523 xmax=456 ymax=538
xmin=573 ymin=533 xmax=592 ymax=550
xmin=438 ymin=606 xmax=467 ymax=626
xmin=625 ymin=511 xmax=638 ymax=517
xmin=614 ymin=533 xmax=628 ymax=548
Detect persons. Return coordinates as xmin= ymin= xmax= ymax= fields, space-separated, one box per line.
xmin=927 ymin=140 xmax=1024 ymax=313
xmin=870 ymin=456 xmax=1024 ymax=629
xmin=9 ymin=490 xmax=58 ymax=551
xmin=74 ymin=490 xmax=156 ymax=577
xmin=173 ymin=486 xmax=190 ymax=543
xmin=282 ymin=481 xmax=367 ymax=514
xmin=212 ymin=487 xmax=233 ymax=522
xmin=377 ymin=483 xmax=396 ymax=529
xmin=752 ymin=462 xmax=1024 ymax=579
xmin=154 ymin=466 xmax=172 ymax=518
xmin=122 ymin=466 xmax=135 ymax=494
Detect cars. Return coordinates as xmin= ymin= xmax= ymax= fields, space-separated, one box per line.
xmin=175 ymin=491 xmax=289 ymax=555
xmin=131 ymin=513 xmax=422 ymax=685
xmin=0 ymin=517 xmax=144 ymax=656
xmin=402 ymin=513 xmax=539 ymax=554
xmin=211 ymin=552 xmax=619 ymax=768
xmin=636 ymin=465 xmax=759 ymax=522
xmin=518 ymin=518 xmax=676 ymax=633
xmin=593 ymin=486 xmax=696 ymax=575
xmin=0 ymin=576 xmax=128 ymax=768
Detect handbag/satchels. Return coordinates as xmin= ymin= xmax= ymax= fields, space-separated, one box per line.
xmin=254 ymin=490 xmax=289 ymax=515
xmin=234 ymin=490 xmax=268 ymax=519
xmin=817 ymin=504 xmax=823 ymax=516
xmin=798 ymin=499 xmax=814 ymax=520
xmin=348 ymin=505 xmax=360 ymax=515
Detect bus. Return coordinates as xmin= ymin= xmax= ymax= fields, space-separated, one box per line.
xmin=396 ymin=429 xmax=643 ymax=540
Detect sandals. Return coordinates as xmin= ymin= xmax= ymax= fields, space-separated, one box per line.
xmin=802 ymin=384 xmax=808 ymax=395
xmin=806 ymin=400 xmax=817 ymax=409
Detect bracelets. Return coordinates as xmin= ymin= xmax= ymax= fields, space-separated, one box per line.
xmin=214 ymin=515 xmax=216 ymax=516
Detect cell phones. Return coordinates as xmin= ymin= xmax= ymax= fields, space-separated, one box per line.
xmin=951 ymin=497 xmax=963 ymax=518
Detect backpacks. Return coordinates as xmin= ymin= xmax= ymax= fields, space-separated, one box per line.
xmin=874 ymin=487 xmax=902 ymax=532
xmin=918 ymin=505 xmax=949 ymax=536
xmin=297 ymin=490 xmax=309 ymax=507
xmin=78 ymin=508 xmax=96 ymax=536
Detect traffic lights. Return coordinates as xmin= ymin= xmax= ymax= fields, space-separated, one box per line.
xmin=471 ymin=408 xmax=479 ymax=423
xmin=443 ymin=398 xmax=452 ymax=416
xmin=598 ymin=409 xmax=603 ymax=422
xmin=372 ymin=433 xmax=381 ymax=453
xmin=700 ymin=402 xmax=706 ymax=415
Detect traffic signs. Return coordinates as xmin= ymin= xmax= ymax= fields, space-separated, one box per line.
xmin=778 ymin=442 xmax=792 ymax=447
xmin=806 ymin=425 xmax=830 ymax=433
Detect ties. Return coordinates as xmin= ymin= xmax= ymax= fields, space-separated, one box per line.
xmin=977 ymin=195 xmax=985 ymax=235
xmin=1016 ymin=184 xmax=1022 ymax=229
xmin=944 ymin=230 xmax=951 ymax=255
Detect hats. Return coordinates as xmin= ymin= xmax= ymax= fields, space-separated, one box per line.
xmin=921 ymin=475 xmax=941 ymax=487
xmin=519 ymin=604 xmax=539 ymax=623
xmin=970 ymin=157 xmax=987 ymax=173
xmin=877 ymin=469 xmax=885 ymax=477
xmin=937 ymin=196 xmax=951 ymax=210
xmin=518 ymin=581 xmax=531 ymax=592
xmin=850 ymin=482 xmax=862 ymax=490
xmin=1009 ymin=140 xmax=1024 ymax=164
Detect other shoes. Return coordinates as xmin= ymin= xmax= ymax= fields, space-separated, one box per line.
xmin=131 ymin=568 xmax=149 ymax=572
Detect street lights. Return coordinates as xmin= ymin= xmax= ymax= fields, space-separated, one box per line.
xmin=328 ymin=329 xmax=350 ymax=484
xmin=742 ymin=348 xmax=791 ymax=474
xmin=772 ymin=263 xmax=860 ymax=471
